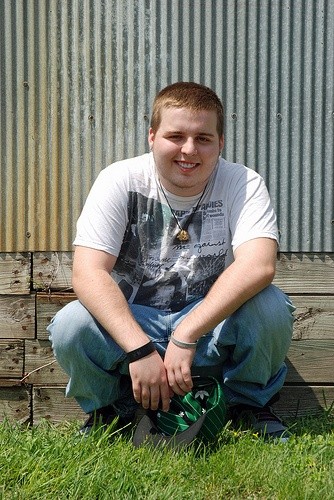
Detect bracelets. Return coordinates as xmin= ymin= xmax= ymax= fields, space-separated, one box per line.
xmin=170 ymin=331 xmax=200 ymax=348
xmin=126 ymin=340 xmax=155 ymax=363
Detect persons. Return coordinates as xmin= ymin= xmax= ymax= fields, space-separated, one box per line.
xmin=47 ymin=81 xmax=296 ymax=445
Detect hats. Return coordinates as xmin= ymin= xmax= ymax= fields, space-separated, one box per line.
xmin=133 ymin=377 xmax=229 ymax=458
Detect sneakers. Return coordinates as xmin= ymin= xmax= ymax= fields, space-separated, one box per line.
xmin=79 ymin=409 xmax=125 ymax=438
xmin=231 ymin=406 xmax=291 ymax=443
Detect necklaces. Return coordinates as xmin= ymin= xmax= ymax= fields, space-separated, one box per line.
xmin=159 ymin=178 xmax=209 ymax=241
